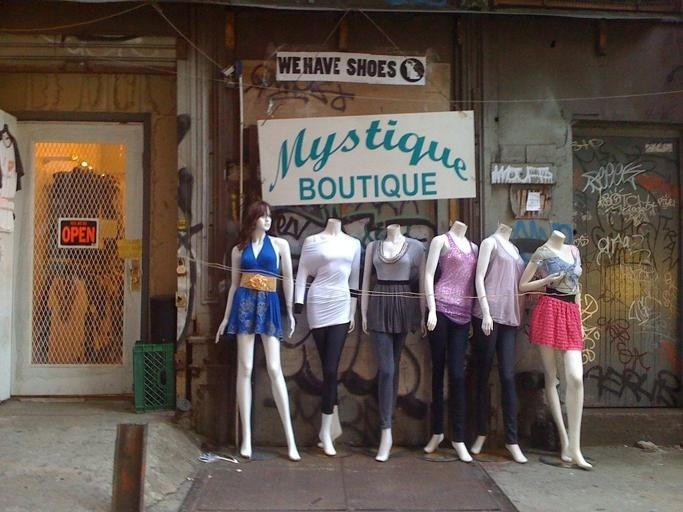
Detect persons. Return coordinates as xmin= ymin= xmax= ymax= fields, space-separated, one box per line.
xmin=519 ymin=229 xmax=593 ymax=470
xmin=470 ymin=224 xmax=528 ymax=463
xmin=424 ymin=219 xmax=478 ymax=462
xmin=361 ymin=222 xmax=426 ymax=461
xmin=214 ymin=200 xmax=302 ymax=462
xmin=293 ymin=219 xmax=361 ymax=457
